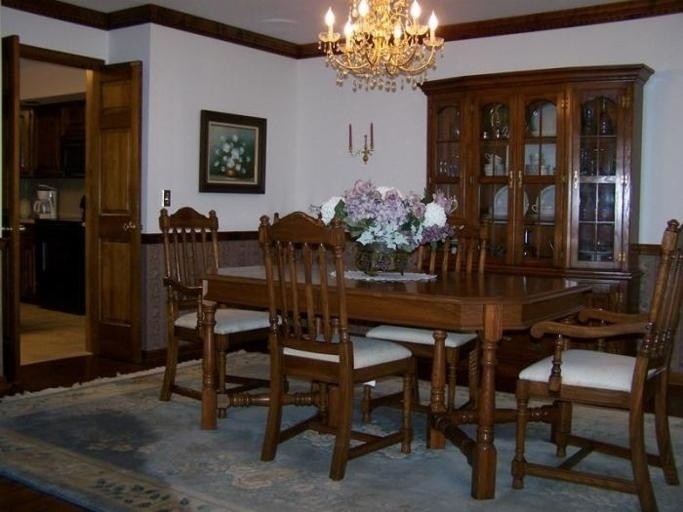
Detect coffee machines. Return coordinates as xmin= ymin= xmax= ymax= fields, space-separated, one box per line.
xmin=32 ymin=183 xmax=58 ymax=220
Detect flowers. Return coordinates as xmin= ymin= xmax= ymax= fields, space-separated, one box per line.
xmin=311 ymin=176 xmax=462 ymax=252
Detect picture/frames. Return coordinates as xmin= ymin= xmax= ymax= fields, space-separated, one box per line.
xmin=197 ymin=109 xmax=267 ymax=195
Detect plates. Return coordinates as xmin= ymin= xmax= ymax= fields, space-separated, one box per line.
xmin=581 ymin=250 xmax=613 ymax=257
xmin=489 ymin=184 xmax=529 ymax=220
xmin=533 ymin=184 xmax=555 ymax=209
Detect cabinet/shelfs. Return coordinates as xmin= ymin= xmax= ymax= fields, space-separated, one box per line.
xmin=19 ymin=220 xmax=37 ymax=299
xmin=19 ymin=101 xmax=34 ymax=178
xmin=415 ymin=61 xmax=655 ymax=274
xmin=33 ymin=97 xmax=85 ymax=180
xmin=416 ymin=273 xmax=640 ymax=388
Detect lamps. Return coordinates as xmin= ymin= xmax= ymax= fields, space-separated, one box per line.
xmin=348 ymin=122 xmax=375 ymax=164
xmin=316 ymin=0 xmax=444 ymax=92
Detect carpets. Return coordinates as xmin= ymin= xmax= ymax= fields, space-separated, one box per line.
xmin=0 ymin=349 xmax=681 ymax=511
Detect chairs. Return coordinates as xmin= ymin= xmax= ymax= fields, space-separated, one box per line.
xmin=157 ymin=206 xmax=287 ymax=418
xmin=258 ymin=212 xmax=414 ymax=481
xmin=512 ymin=220 xmax=683 ymax=512
xmin=362 ymin=215 xmax=490 ymax=424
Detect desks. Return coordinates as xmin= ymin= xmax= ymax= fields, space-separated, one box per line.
xmin=198 ymin=264 xmax=592 ymax=449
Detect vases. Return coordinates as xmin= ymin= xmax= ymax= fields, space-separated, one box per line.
xmin=358 ymin=241 xmax=408 ymax=277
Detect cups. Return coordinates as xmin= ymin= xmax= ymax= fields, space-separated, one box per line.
xmin=531 ymin=203 xmax=554 ymax=216
xmin=485 ymin=164 xmax=503 ymax=176
xmin=483 ymin=150 xmax=503 ymax=164
xmin=524 ymin=152 xmax=550 ymax=175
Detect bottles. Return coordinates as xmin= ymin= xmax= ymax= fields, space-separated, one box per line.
xmin=584 ymin=99 xmax=613 ymax=134
xmin=580 ymin=148 xmax=594 ymax=176
